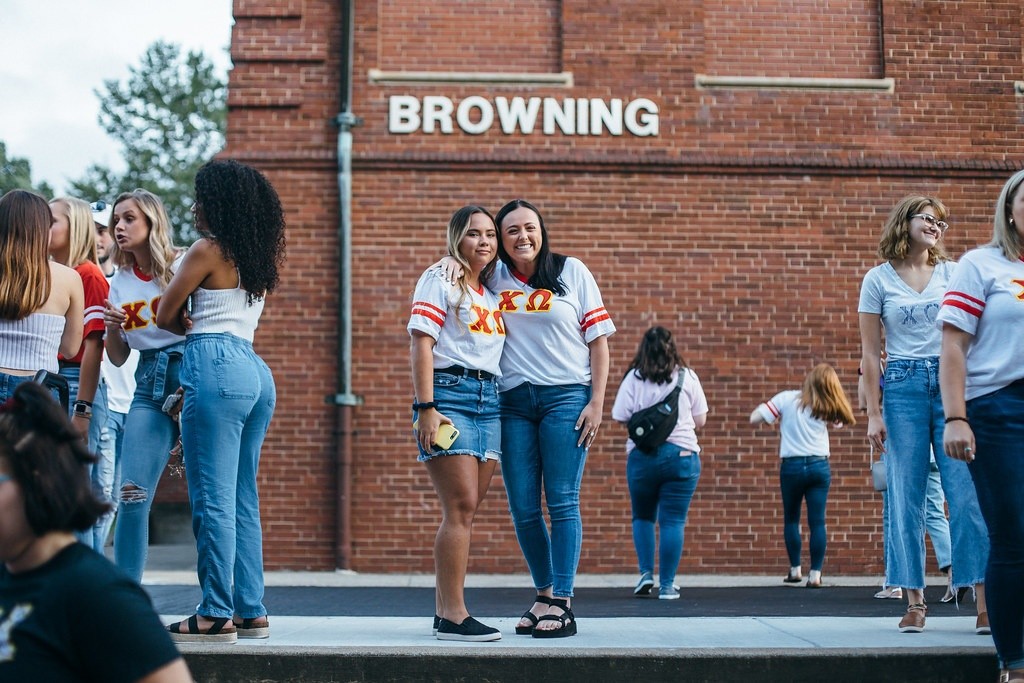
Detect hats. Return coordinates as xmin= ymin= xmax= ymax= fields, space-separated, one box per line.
xmin=90 ymin=200 xmax=112 ymax=225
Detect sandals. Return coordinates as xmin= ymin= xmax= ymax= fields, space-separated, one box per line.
xmin=939 ymin=579 xmax=969 ymax=603
xmin=515 ymin=596 xmax=578 ymax=638
xmin=874 ymin=586 xmax=904 ymax=599
xmin=164 ymin=611 xmax=271 ymax=643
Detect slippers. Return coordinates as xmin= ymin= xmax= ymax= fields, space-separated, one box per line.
xmin=783 ymin=570 xmax=803 ymax=582
xmin=807 ymin=575 xmax=823 ymax=588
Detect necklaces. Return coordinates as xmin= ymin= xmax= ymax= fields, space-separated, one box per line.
xmin=137 ymin=263 xmax=150 ymax=271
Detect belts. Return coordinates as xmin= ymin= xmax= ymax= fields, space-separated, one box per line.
xmin=434 ymin=366 xmax=492 ymax=381
xmin=783 ymin=455 xmax=827 ymax=462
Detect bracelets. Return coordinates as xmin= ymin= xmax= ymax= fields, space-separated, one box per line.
xmin=858 ymin=367 xmax=863 ymax=375
xmin=411 ymin=401 xmax=438 ymax=412
xmin=943 ymin=416 xmax=969 ymax=424
xmin=73 ymin=400 xmax=93 ymax=408
xmin=73 ymin=411 xmax=91 ymax=421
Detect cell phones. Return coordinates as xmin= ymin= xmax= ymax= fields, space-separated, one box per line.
xmin=186 ymin=296 xmax=192 ymax=320
xmin=413 ymin=418 xmax=460 ymax=450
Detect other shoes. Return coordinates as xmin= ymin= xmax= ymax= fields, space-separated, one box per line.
xmin=433 ymin=613 xmax=502 ymax=641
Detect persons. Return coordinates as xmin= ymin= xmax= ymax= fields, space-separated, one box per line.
xmin=748 ymin=362 xmax=857 ymax=588
xmin=156 ymin=159 xmax=288 ymax=643
xmin=103 ymin=188 xmax=191 ymax=584
xmin=428 ymin=197 xmax=620 ymax=641
xmin=612 ymin=325 xmax=709 ymax=600
xmin=858 ymin=194 xmax=991 ymax=635
xmin=858 ymin=350 xmax=969 ymax=604
xmin=0 ymin=188 xmax=140 ymax=555
xmin=0 ymin=381 xmax=196 ymax=683
xmin=935 ymin=169 xmax=1024 ymax=683
xmin=401 ymin=202 xmax=503 ymax=640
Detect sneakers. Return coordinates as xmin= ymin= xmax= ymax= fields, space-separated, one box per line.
xmin=658 ymin=588 xmax=680 ymax=600
xmin=898 ymin=605 xmax=927 ymax=633
xmin=634 ymin=573 xmax=654 ymax=595
xmin=976 ymin=612 xmax=992 ymax=634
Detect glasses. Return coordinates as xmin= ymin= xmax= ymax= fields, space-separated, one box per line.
xmin=96 ymin=199 xmax=106 ymax=210
xmin=911 ymin=214 xmax=949 ymax=231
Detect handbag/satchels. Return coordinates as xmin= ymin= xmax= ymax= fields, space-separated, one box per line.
xmin=625 ymin=365 xmax=687 ymax=454
xmin=871 ymin=461 xmax=888 ymax=491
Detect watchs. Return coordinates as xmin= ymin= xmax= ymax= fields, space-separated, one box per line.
xmin=73 ymin=403 xmax=92 ymax=413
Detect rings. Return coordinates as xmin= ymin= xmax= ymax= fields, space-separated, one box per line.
xmin=965 ymin=447 xmax=971 ymax=451
xmin=588 ymin=431 xmax=595 ymax=436
